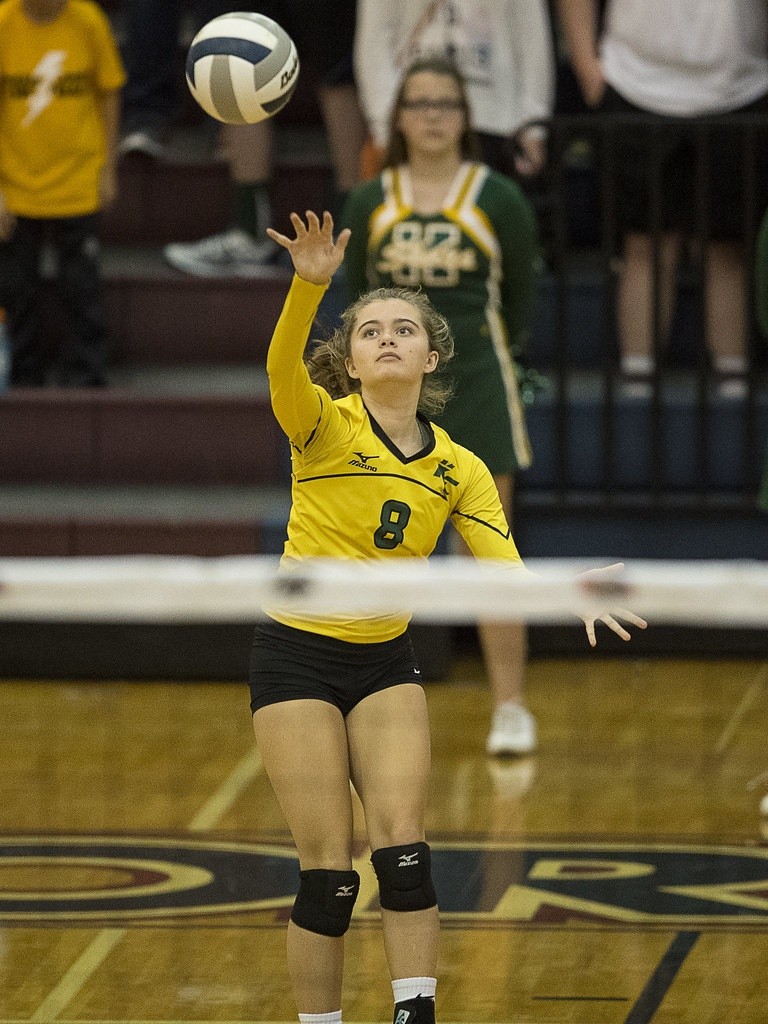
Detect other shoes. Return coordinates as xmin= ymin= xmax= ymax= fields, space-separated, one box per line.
xmin=621 ymin=353 xmax=654 ymax=396
xmin=718 ymin=357 xmax=748 ymax=398
xmin=487 ymin=758 xmax=537 ymax=799
xmin=485 ymin=704 xmax=536 ymax=755
xmin=164 ymin=227 xmax=279 ymax=279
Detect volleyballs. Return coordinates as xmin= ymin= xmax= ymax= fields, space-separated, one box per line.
xmin=185 ymin=11 xmax=299 ymax=125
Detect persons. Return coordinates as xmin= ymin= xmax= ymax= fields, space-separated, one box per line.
xmin=249 ymin=211 xmax=660 ymax=1024
xmin=331 ymin=0 xmax=768 ymax=411
xmin=0 ymin=0 xmax=127 ymax=387
xmin=334 ymin=60 xmax=547 ymax=756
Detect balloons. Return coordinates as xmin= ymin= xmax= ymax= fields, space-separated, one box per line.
xmin=185 ymin=11 xmax=302 ymax=125
